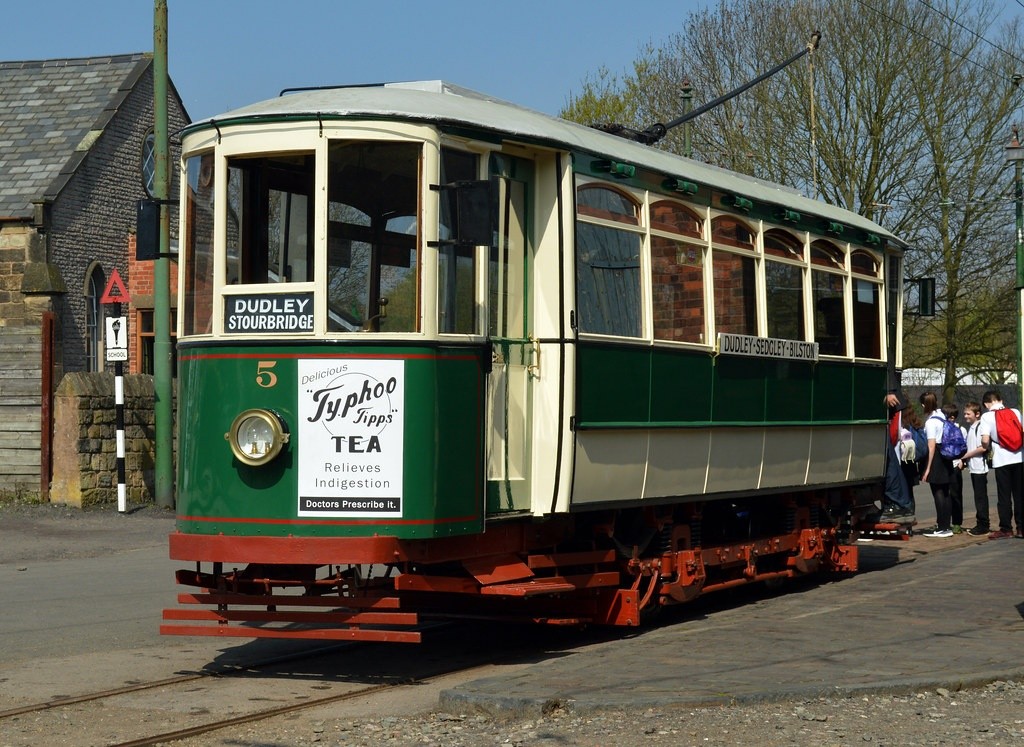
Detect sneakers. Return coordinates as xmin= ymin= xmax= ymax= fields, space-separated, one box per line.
xmin=1016 ymin=529 xmax=1024 ymax=538
xmin=902 ymin=506 xmax=916 ymax=523
xmin=952 ymin=525 xmax=961 ymax=534
xmin=864 ymin=501 xmax=905 ymax=523
xmin=988 ymin=528 xmax=1013 ymax=540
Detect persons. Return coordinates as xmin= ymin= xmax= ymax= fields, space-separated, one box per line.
xmin=880 ymin=348 xmax=1024 ymax=540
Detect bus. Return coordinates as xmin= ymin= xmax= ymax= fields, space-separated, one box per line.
xmin=156 ymin=78 xmax=936 ymax=644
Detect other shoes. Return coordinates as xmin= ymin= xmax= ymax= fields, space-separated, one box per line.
xmin=922 ymin=527 xmax=953 ymax=538
xmin=966 ymin=525 xmax=990 ymax=536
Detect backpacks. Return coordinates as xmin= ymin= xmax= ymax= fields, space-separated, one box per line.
xmin=989 ymin=408 xmax=1024 ymax=453
xmin=930 ymin=415 xmax=967 ymax=459
xmin=899 ymin=428 xmax=915 ymax=461
xmin=909 ymin=424 xmax=928 ymax=460
